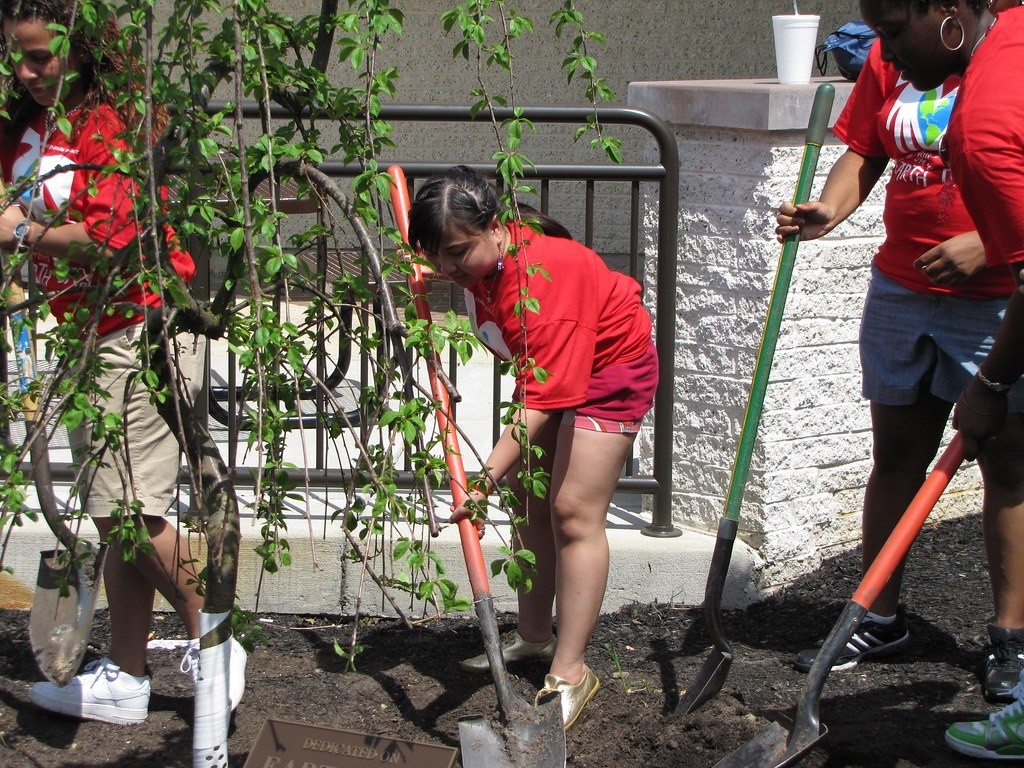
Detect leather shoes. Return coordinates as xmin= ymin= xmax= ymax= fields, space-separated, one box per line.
xmin=533 ymin=663 xmax=600 ymax=733
xmin=461 ymin=629 xmax=558 ymax=671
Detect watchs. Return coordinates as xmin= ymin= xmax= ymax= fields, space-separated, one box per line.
xmin=14 ymin=218 xmax=32 ymax=248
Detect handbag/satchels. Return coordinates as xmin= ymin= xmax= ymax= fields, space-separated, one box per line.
xmin=814 ymin=20 xmax=878 ymax=82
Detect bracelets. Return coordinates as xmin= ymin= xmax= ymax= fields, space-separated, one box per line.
xmin=471 ymin=483 xmax=491 ymax=496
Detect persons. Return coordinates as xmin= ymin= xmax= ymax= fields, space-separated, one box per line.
xmin=776 ymin=0 xmax=1023 ymax=761
xmin=1 ymin=1 xmax=247 ymax=726
xmin=406 ymin=165 xmax=660 ymax=733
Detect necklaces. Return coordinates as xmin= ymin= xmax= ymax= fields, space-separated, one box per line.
xmin=480 ymin=274 xmax=499 ymax=303
xmin=970 ymin=16 xmax=998 ymax=58
xmin=44 ymin=102 xmax=84 ymax=140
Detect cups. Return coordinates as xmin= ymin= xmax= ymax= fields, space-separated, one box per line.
xmin=772 ymin=14 xmax=821 ymax=84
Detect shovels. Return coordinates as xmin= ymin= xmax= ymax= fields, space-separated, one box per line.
xmin=711 ymin=428 xmax=966 ymax=768
xmin=386 ymin=165 xmax=566 ymax=768
xmin=0 ymin=175 xmax=111 ymax=689
xmin=673 ymin=83 xmax=835 ymax=716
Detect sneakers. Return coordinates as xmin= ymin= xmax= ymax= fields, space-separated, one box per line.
xmin=793 ymin=604 xmax=910 ymax=672
xmin=980 ymin=624 xmax=1024 ymax=701
xmin=179 ymin=638 xmax=247 ymax=712
xmin=28 ymin=657 xmax=151 ymax=726
xmin=944 ymin=668 xmax=1024 ymax=759
